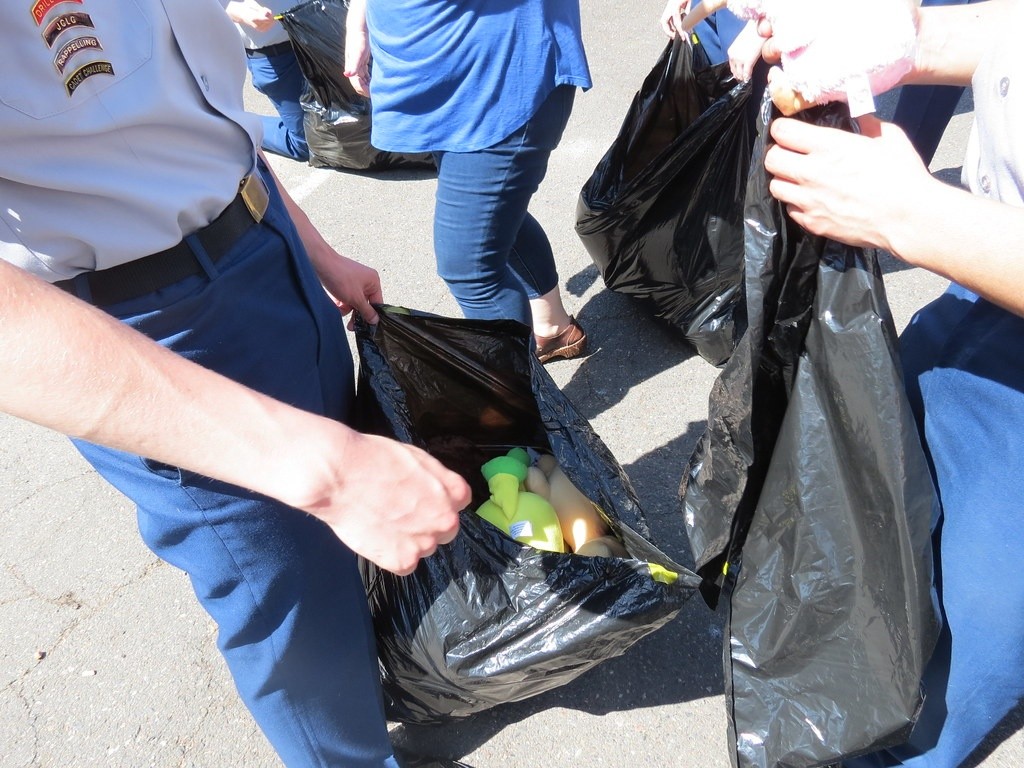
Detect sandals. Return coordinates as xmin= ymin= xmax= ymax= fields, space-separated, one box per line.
xmin=535 ymin=315 xmax=586 ymax=363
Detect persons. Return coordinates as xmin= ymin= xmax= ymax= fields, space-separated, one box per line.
xmin=339 ymin=0 xmax=596 ymax=367
xmin=745 ymin=8 xmax=1024 ymax=767
xmin=657 ymin=0 xmax=770 ymax=86
xmin=216 ymin=1 xmax=317 ymax=165
xmin=2 ymin=0 xmax=472 ymax=767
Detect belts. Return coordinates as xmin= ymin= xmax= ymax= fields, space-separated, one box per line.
xmin=52 ymin=172 xmax=270 ymax=308
